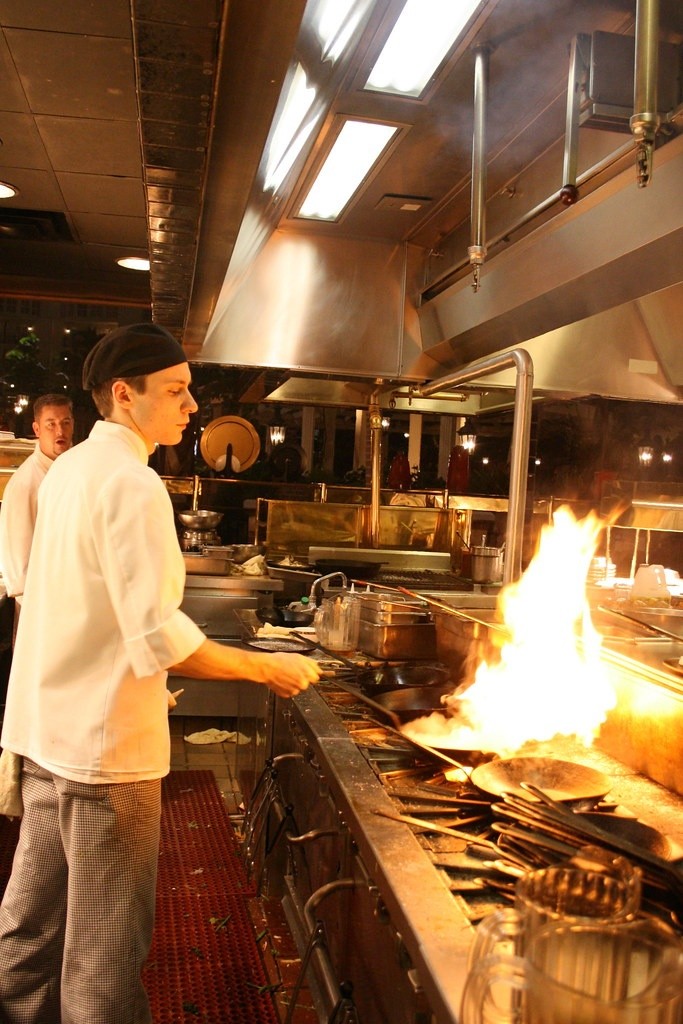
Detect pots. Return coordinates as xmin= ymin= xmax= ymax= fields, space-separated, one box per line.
xmin=226 ymin=544 xmax=265 ymax=566
xmin=455 ymin=531 xmax=505 ymax=584
xmin=175 ymin=508 xmax=224 ymax=532
xmin=272 ymin=662 xmax=670 ymax=904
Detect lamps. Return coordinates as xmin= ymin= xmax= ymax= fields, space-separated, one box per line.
xmin=268 ymin=406 xmax=287 ymax=447
xmin=14 ymin=387 xmax=32 ymax=417
xmin=381 ymin=411 xmax=392 ymax=431
xmin=457 ymin=415 xmax=478 ymax=451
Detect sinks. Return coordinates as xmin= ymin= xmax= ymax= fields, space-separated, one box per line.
xmin=175 ymin=509 xmax=224 ymax=530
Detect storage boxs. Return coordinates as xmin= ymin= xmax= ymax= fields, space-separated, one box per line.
xmin=359 ymin=593 xmax=438 ymax=660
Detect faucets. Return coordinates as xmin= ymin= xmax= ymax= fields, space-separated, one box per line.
xmin=191 ymin=475 xmax=202 ymax=512
xmin=309 ymin=572 xmax=348 ymax=604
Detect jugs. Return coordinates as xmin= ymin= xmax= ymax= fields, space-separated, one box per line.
xmin=314 ymin=596 xmax=362 ymax=654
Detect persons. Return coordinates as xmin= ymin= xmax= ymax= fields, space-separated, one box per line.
xmin=0 ymin=322 xmax=328 ymax=1024
xmin=0 ymin=395 xmax=75 ymax=893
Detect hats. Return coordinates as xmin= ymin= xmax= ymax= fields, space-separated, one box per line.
xmin=84 ymin=324 xmax=187 ymax=389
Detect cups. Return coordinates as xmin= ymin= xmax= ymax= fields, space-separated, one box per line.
xmin=467 ymin=866 xmax=642 ymax=1024
xmin=459 ymin=917 xmax=683 ymax=1024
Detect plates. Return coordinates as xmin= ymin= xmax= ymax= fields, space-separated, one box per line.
xmin=588 ymin=561 xmax=618 ymax=585
